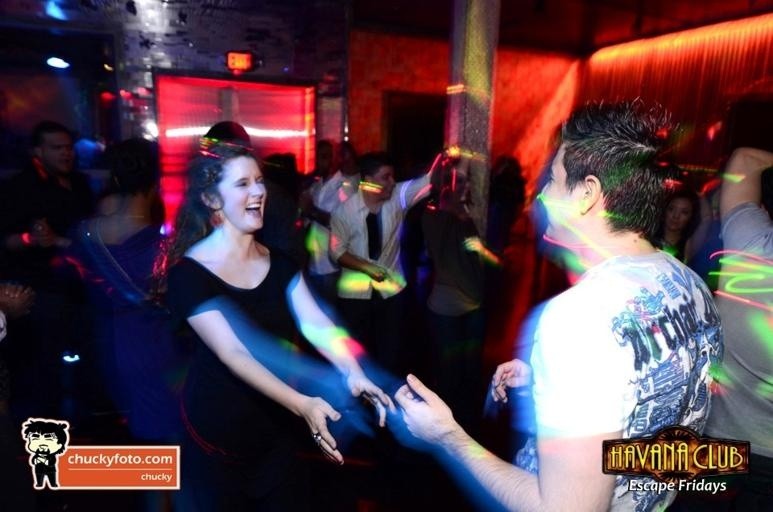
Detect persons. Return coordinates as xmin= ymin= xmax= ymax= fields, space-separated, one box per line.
xmin=0 ymin=87 xmax=772 ymax=512
